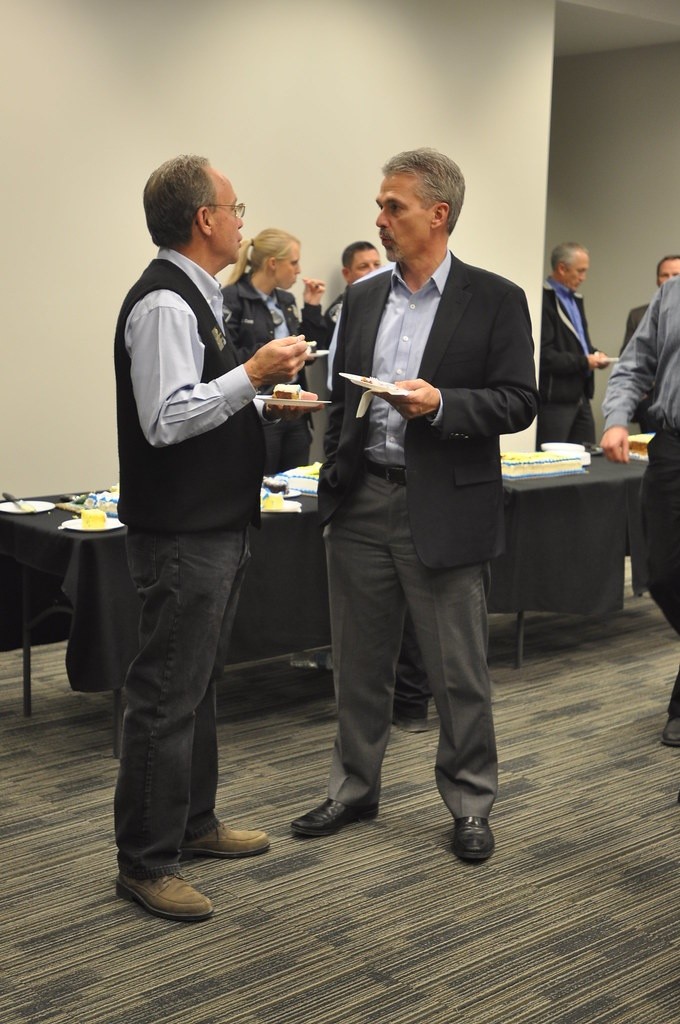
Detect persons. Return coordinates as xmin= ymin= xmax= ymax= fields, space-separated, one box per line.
xmin=291 ymin=148 xmax=536 ymax=863
xmin=115 ymin=156 xmax=313 ymax=920
xmin=219 ymin=228 xmax=680 ymax=747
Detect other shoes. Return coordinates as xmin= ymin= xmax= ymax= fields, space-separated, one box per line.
xmin=392 ymin=713 xmax=428 ymax=732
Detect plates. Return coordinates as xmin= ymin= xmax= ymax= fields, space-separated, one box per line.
xmin=263 ymin=500 xmax=302 ymax=512
xmin=284 ymin=489 xmax=302 ymax=498
xmin=0 ymin=500 xmax=56 ymax=514
xmin=338 ymin=372 xmax=408 ymax=394
xmin=540 ymin=442 xmax=585 ymax=454
xmin=305 ymin=350 xmax=330 ymax=357
xmin=254 ymin=394 xmax=332 ymax=407
xmin=62 ymin=517 xmax=126 ymax=532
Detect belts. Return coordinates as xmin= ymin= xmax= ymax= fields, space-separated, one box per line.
xmin=662 ymin=422 xmax=680 ymax=437
xmin=367 ymin=458 xmax=407 ymax=486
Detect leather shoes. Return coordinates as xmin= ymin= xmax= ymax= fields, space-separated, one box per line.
xmin=452 ymin=816 xmax=495 ymax=863
xmin=290 ymin=798 xmax=379 ymax=837
xmin=181 ymin=823 xmax=270 ymax=860
xmin=116 ymin=872 xmax=215 ymax=921
xmin=661 ymin=714 xmax=680 ymax=746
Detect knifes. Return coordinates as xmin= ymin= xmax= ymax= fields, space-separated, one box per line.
xmin=2 ymin=493 xmax=37 ymax=510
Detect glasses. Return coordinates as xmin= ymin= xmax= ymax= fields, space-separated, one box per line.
xmin=195 ymin=202 xmax=246 ymax=225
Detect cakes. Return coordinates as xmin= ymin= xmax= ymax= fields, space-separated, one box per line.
xmin=259 ymin=463 xmax=322 ymax=508
xmin=71 ymin=483 xmax=120 ymax=529
xmin=273 ymin=383 xmax=301 ymax=400
xmin=499 ymin=451 xmax=586 ymax=478
xmin=625 ymin=432 xmax=656 ymax=456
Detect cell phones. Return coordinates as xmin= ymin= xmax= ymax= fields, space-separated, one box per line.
xmin=601 ymin=358 xmax=620 ymax=362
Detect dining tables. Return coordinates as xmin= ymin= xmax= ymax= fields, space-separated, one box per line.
xmin=0 ymin=442 xmax=654 ymax=757
